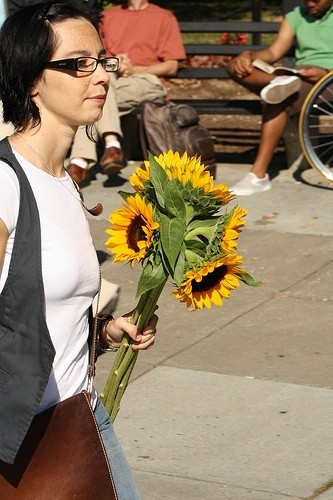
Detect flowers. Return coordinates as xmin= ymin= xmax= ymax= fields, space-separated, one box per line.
xmin=99 ymin=148 xmax=264 ymax=423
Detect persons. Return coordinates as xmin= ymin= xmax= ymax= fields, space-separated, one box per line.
xmin=0 ymin=0 xmax=158 ymax=500
xmin=64 ymin=0 xmax=186 ymax=185
xmin=228 ymin=0 xmax=333 ymax=196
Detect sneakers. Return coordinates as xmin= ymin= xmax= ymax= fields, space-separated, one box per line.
xmin=261 ymin=75 xmax=301 ymax=103
xmin=228 ymin=172 xmax=271 ymax=196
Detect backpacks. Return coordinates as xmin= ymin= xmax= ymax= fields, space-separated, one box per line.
xmin=144 ymin=101 xmax=215 ymax=182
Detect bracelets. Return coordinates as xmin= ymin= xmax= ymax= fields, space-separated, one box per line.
xmin=97 ymin=314 xmax=118 ymax=353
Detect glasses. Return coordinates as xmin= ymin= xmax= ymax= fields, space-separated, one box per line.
xmin=43 ymin=56 xmax=119 ymax=73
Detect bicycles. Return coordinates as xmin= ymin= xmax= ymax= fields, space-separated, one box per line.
xmin=298 ymin=68 xmax=333 ymax=187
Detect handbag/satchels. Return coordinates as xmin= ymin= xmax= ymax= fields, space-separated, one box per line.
xmin=0 ymin=392 xmax=119 ymax=500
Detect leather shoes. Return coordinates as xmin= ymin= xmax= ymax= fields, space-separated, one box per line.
xmin=100 ymin=145 xmax=128 ymax=174
xmin=66 ymin=163 xmax=91 ymax=186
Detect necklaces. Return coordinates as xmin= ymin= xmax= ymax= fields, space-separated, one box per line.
xmin=18 ymin=134 xmax=81 ymax=201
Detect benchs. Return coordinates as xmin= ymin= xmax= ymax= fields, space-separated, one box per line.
xmin=121 ymin=20 xmax=333 ymax=173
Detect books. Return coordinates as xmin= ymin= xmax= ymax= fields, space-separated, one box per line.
xmin=252 ymin=58 xmax=299 ymax=76
